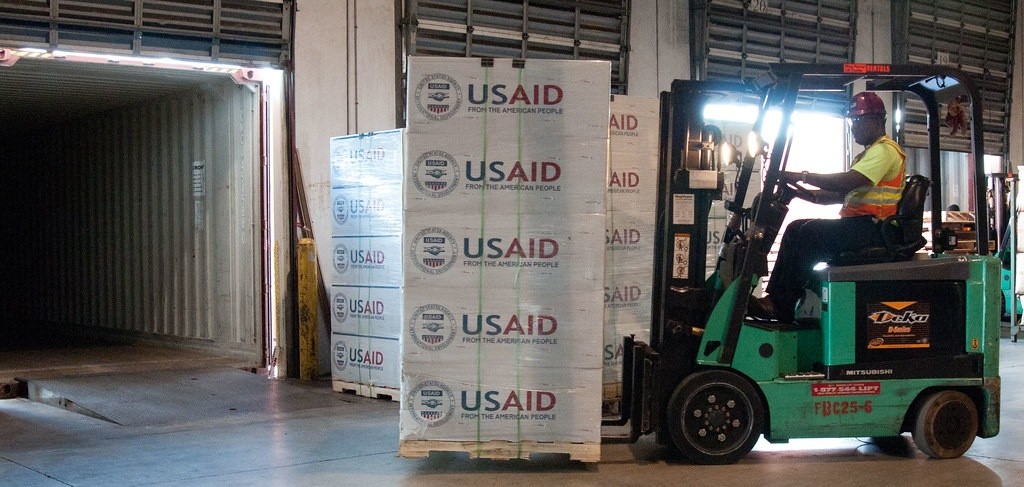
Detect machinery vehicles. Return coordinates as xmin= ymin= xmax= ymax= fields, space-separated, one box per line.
xmin=398 ymin=58 xmax=1010 ymax=467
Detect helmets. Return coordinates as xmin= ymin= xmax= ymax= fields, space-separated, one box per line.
xmin=847 ymin=92 xmax=887 ymax=117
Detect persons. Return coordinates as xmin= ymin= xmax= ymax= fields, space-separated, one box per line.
xmin=752 ymin=92 xmax=907 ymax=324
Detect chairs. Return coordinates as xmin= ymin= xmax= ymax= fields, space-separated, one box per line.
xmin=827 ymin=173 xmax=931 ymax=267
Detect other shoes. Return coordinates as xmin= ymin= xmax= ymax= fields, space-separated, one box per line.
xmin=747 ymin=292 xmax=794 ymax=323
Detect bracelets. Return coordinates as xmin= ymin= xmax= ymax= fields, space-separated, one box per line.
xmin=801 ymin=171 xmax=808 ymax=183
